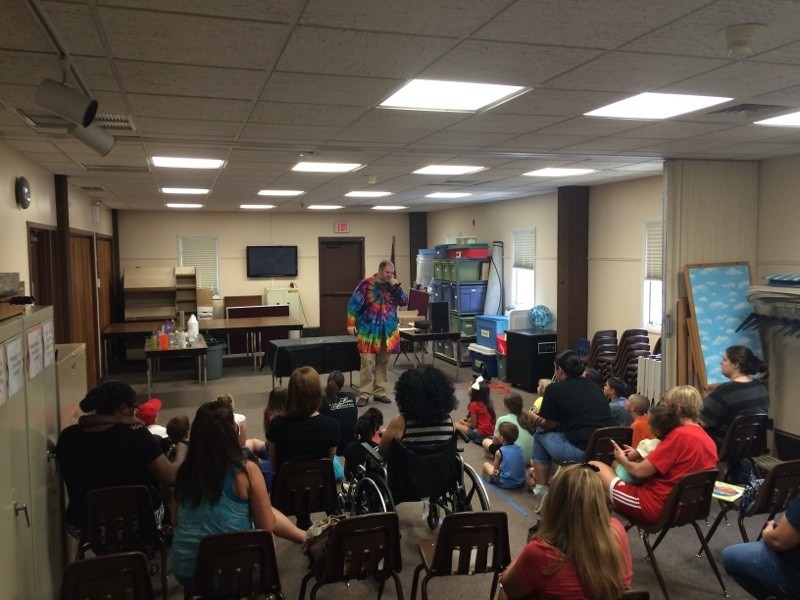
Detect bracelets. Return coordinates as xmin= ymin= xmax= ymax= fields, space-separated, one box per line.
xmin=535 ymin=417 xmax=544 ymax=425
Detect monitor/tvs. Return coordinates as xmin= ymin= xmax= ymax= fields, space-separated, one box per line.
xmin=246 ymin=246 xmax=298 ymax=278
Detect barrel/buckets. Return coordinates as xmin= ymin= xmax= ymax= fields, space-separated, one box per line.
xmin=192 ymin=340 xmax=227 ymax=380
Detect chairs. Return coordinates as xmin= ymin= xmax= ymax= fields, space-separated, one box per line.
xmin=574 ymin=326 xmax=662 ymax=403
xmin=57 ymin=458 xmax=512 ymax=600
xmin=557 ymin=413 xmax=800 ymax=600
xmin=391 ymin=308 xmax=425 ymax=366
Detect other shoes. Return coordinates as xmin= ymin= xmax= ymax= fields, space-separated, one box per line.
xmin=373 ymin=395 xmax=391 ymax=403
xmin=357 ymin=398 xmax=368 ymax=406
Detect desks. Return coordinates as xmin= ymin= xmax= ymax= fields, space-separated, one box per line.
xmin=100 ymin=315 xmax=304 ymax=377
xmin=393 ymin=330 xmax=462 ymax=383
xmin=262 ymin=335 xmax=361 ymax=390
xmin=144 ymin=332 xmax=209 ymax=389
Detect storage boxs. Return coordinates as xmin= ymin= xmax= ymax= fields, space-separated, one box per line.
xmin=416 ymin=235 xmax=533 ymax=380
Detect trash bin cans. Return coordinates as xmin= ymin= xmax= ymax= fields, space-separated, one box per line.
xmin=193 ymin=338 xmax=226 ymax=380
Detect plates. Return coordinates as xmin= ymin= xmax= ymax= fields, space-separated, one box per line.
xmin=528 ymin=305 xmax=552 ymax=328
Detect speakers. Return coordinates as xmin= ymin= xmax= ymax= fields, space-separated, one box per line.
xmin=428 ymin=301 xmax=450 ymax=333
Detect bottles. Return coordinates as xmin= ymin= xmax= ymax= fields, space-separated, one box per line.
xmin=150 ymin=318 xmax=186 ymax=350
xmin=187 ymin=313 xmax=199 ymax=338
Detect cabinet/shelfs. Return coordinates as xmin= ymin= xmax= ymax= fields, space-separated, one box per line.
xmin=121 ymin=266 xmax=198 ymax=322
xmin=0 ymin=306 xmax=88 ymax=600
xmin=432 ymin=257 xmax=492 ymax=368
xmin=503 ymin=330 xmax=557 ymax=392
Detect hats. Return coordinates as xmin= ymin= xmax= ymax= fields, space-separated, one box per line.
xmin=136 ymin=398 xmax=160 ymax=421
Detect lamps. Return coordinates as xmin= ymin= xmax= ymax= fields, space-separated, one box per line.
xmin=34 ymin=56 xmax=98 ymax=128
xmin=92 ymin=200 xmax=101 ymax=223
xmin=64 ymin=118 xmax=117 ymax=158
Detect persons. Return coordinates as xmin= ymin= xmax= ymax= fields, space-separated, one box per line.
xmin=169 ymin=400 xmax=333 ymax=588
xmin=217 ymin=393 xmax=246 ymax=448
xmin=528 ymin=351 xmax=613 ymax=513
xmin=378 ymin=366 xmax=458 ymax=459
xmin=454 ymin=377 xmax=496 ymax=442
xmin=604 ymin=377 xmax=632 ymax=427
xmin=499 ymin=464 xmax=631 ymax=600
xmin=136 ymin=398 xmax=190 ymax=461
xmin=346 ymin=260 xmax=409 ymax=406
xmin=625 ymin=394 xmax=656 ymax=448
xmin=56 ymin=382 xmax=189 ymax=548
xmin=483 ymin=391 xmax=533 ymax=468
xmin=699 ymin=346 xmax=767 ymax=452
xmin=483 ymin=422 xmax=527 ymax=489
xmin=611 ymin=405 xmax=679 ymax=484
xmin=588 ymin=385 xmax=718 ymax=525
xmin=720 ymin=494 xmax=800 ymax=600
xmin=533 ymin=379 xmax=553 ymax=411
xmin=247 ymin=366 xmax=358 ymax=515
